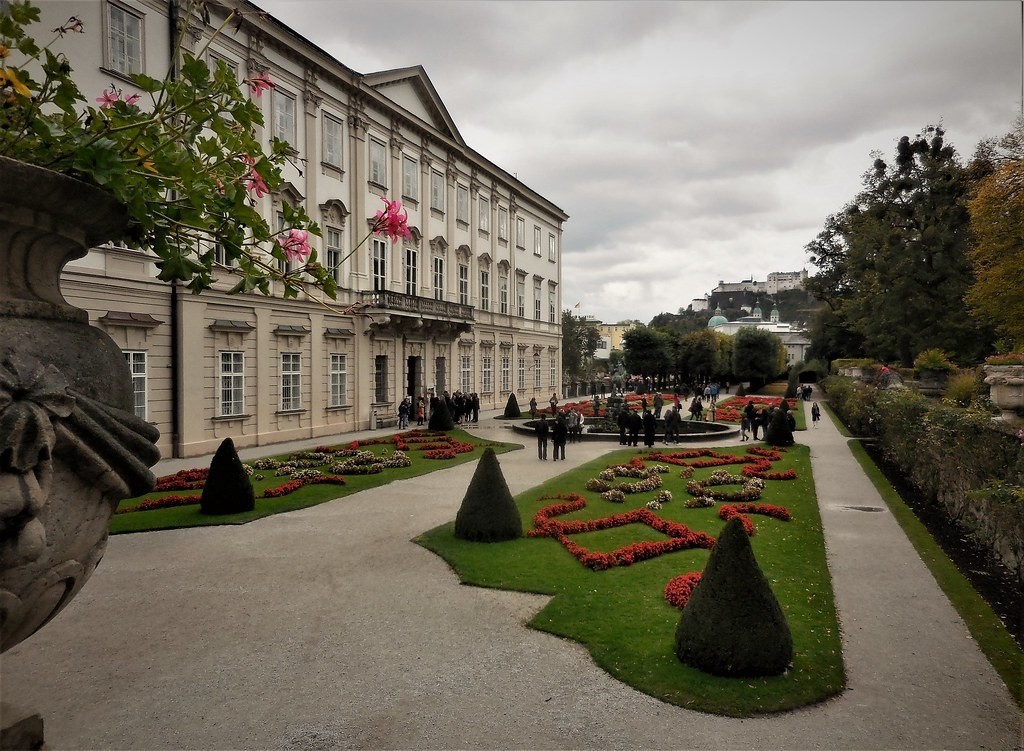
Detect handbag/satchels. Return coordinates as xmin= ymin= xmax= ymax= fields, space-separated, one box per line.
xmin=678 ymin=404 xmax=682 ymax=410
xmin=688 ymin=407 xmax=693 ymax=412
xmin=579 ymin=424 xmax=584 ymax=431
xmin=706 ymin=410 xmax=713 ymax=421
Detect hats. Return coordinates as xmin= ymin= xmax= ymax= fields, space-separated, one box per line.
xmin=749 ymin=400 xmax=755 ymax=404
xmin=780 ymin=400 xmax=791 ymax=411
xmin=740 ymin=412 xmax=747 ymax=417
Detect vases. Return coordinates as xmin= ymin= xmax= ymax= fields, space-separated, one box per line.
xmin=1 ymin=153 xmax=161 ymax=655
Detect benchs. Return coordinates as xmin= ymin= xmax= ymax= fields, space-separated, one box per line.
xmin=376 ymin=413 xmax=399 ymax=429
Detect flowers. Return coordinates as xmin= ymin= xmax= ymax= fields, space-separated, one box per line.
xmin=0 ymin=0 xmax=413 ymax=319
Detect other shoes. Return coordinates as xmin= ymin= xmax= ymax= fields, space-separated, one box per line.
xmin=746 ymin=437 xmax=749 ymax=441
xmin=753 ymin=438 xmax=760 ymax=441
xmin=666 ymin=442 xmax=668 ymax=445
xmin=740 ymin=439 xmax=744 ymax=441
xmin=812 ymin=425 xmax=815 ymax=427
xmin=633 ymin=444 xmax=637 ymax=447
xmin=815 ymin=427 xmax=818 ymax=429
xmin=676 ymin=443 xmax=682 ymax=446
xmin=628 ymin=444 xmax=631 ymax=446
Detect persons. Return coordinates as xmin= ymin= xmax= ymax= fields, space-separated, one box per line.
xmin=551 ymin=417 xmax=567 ymax=460
xmin=397 ymin=376 xmax=820 ymax=448
xmin=536 ymin=413 xmax=549 ymax=460
xmin=881 ymin=362 xmax=890 ymax=374
